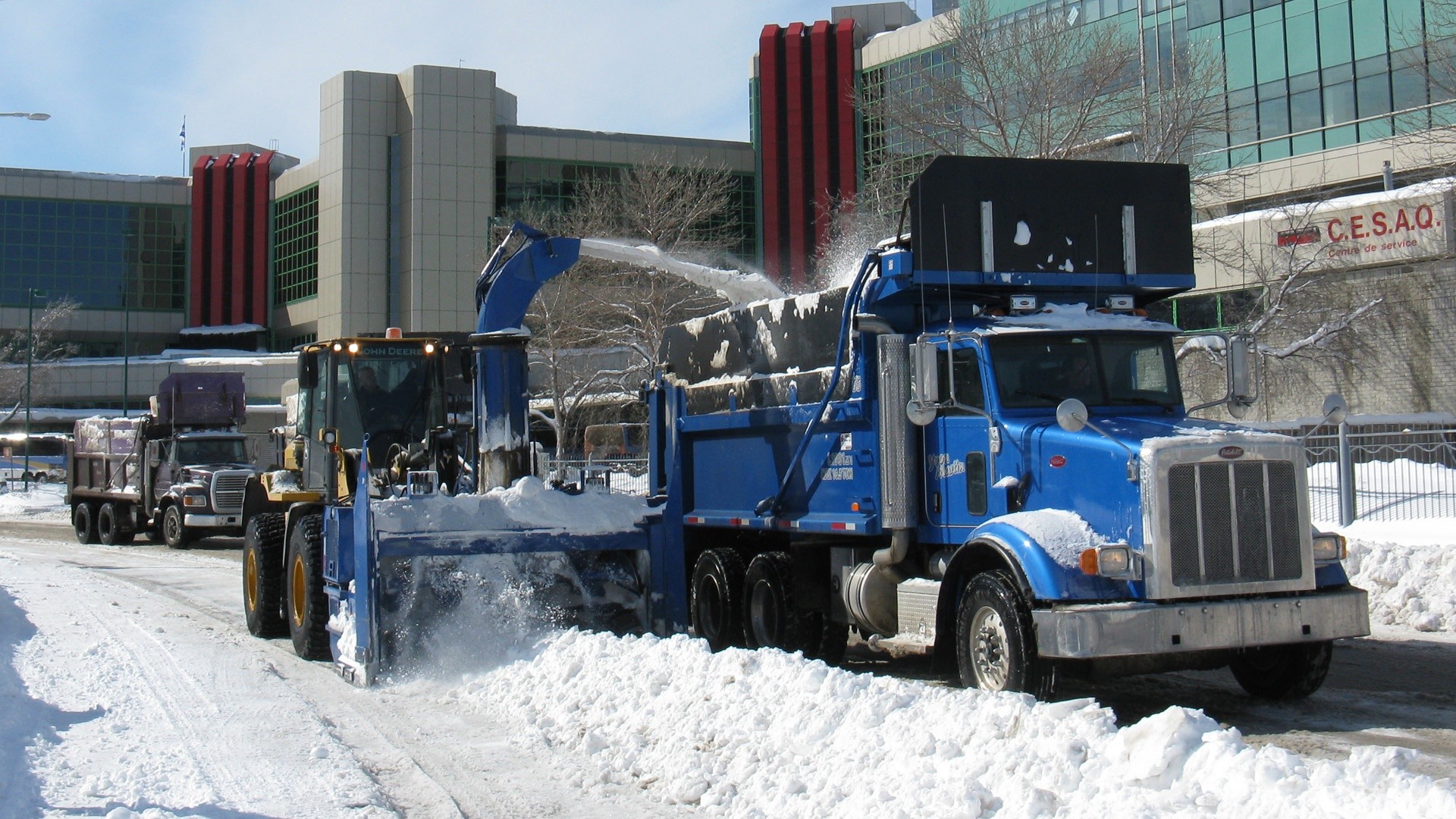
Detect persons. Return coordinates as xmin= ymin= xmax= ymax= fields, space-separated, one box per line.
xmin=1063 ymin=356 xmax=1091 ymax=389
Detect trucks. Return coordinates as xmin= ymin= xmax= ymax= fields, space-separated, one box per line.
xmin=61 ymin=369 xmax=265 ymax=550
xmin=636 ymin=153 xmax=1369 ymax=780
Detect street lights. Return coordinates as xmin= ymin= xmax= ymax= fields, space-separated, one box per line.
xmin=25 ymin=287 xmax=49 ymax=491
xmin=123 ymin=233 xmax=143 ymax=417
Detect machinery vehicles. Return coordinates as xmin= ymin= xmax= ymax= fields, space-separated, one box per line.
xmin=234 ymin=220 xmax=783 ymax=680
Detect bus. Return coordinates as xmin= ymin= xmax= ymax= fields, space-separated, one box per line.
xmin=1 ymin=434 xmax=71 ymax=484
